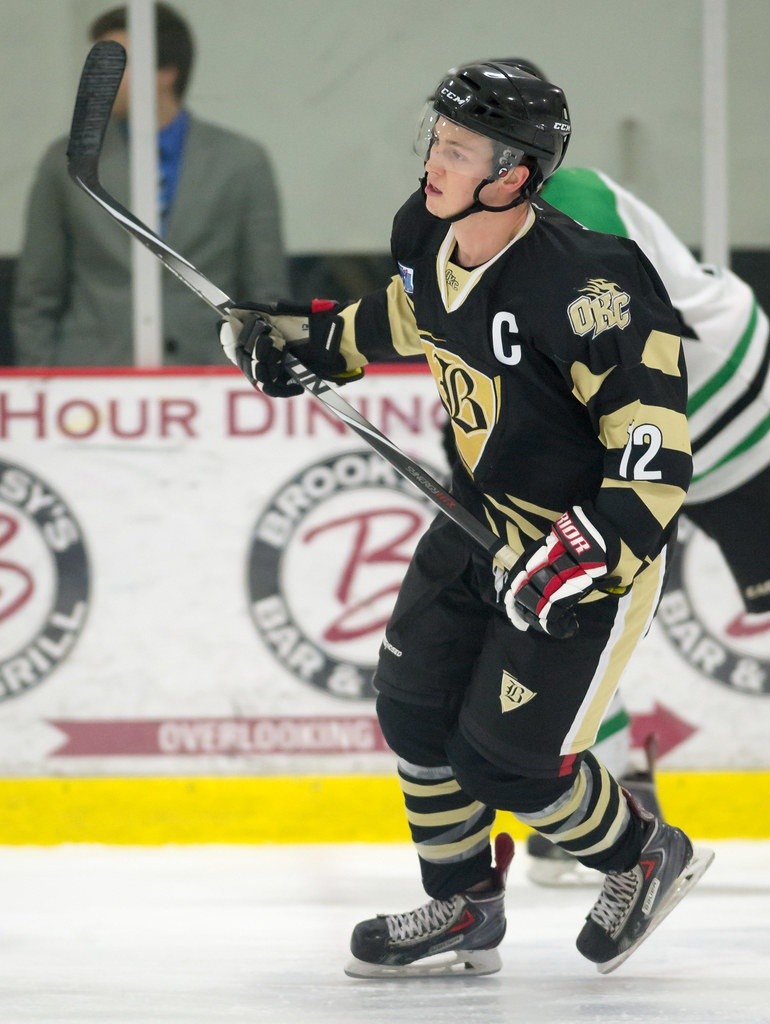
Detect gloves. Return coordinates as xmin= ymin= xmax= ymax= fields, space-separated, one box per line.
xmin=497 ymin=506 xmax=622 ymax=638
xmin=216 ymin=298 xmax=369 ymax=397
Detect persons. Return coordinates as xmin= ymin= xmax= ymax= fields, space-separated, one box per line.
xmin=527 ymin=166 xmax=770 ymax=890
xmin=9 ymin=3 xmax=294 ymax=375
xmin=215 ymin=59 xmax=713 ymax=979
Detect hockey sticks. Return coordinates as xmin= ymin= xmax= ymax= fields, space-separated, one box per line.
xmin=66 ymin=38 xmax=520 ymax=571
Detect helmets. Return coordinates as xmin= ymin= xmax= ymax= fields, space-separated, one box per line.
xmin=433 ymin=62 xmax=572 ymax=195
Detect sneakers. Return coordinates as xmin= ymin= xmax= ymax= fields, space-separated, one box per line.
xmin=344 ymin=833 xmax=515 ymax=978
xmin=577 ymin=786 xmax=715 ymax=974
xmin=528 ymin=732 xmax=661 ymax=887
